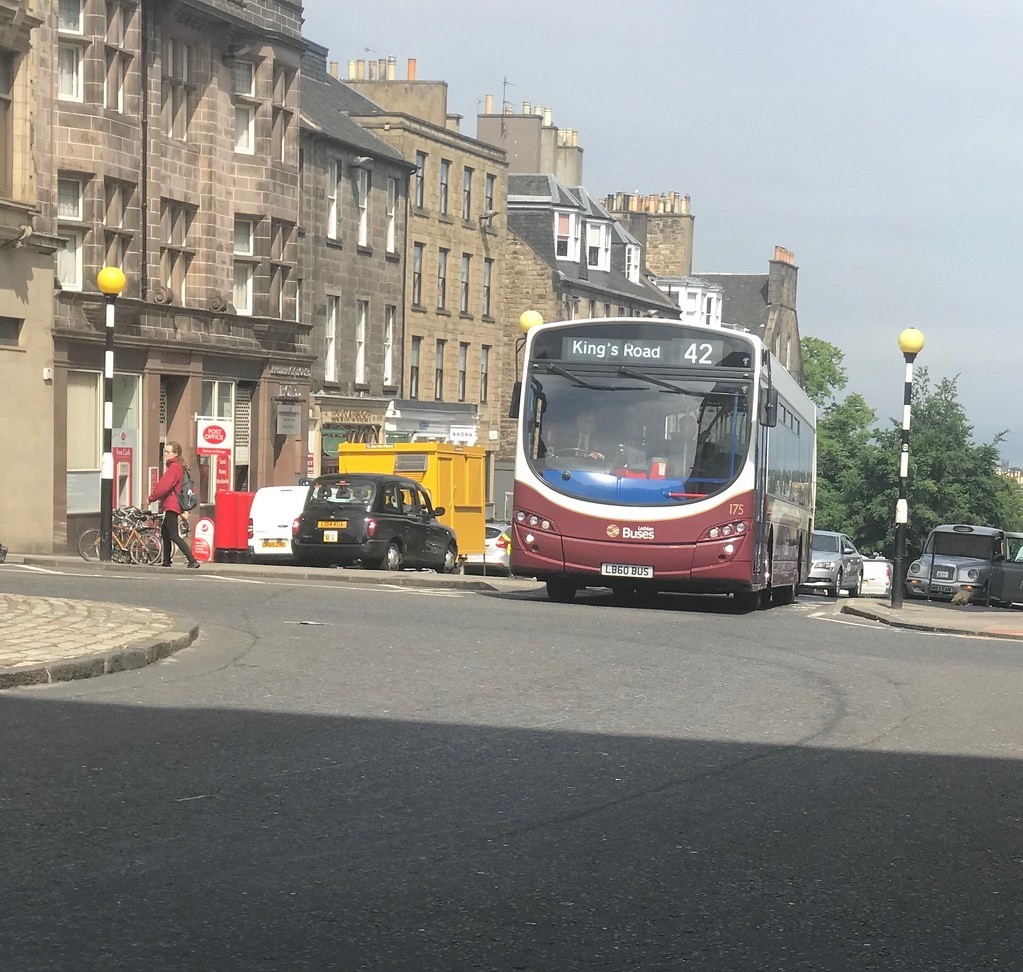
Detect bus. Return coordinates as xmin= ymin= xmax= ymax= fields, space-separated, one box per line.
xmin=508 ymin=317 xmax=817 ymax=613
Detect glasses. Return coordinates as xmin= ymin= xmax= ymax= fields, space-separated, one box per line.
xmin=165 ymin=450 xmax=177 ymax=455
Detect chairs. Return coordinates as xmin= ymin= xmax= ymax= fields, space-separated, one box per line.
xmin=349 ymin=488 xmax=370 ymax=503
xmin=318 ymin=486 xmax=332 ymax=500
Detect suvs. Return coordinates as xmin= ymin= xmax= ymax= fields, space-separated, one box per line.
xmin=905 ymin=524 xmax=1023 ymax=607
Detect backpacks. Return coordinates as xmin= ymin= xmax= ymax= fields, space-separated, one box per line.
xmin=173 ymin=466 xmax=198 ymax=511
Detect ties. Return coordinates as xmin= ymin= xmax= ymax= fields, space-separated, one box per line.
xmin=577 ymin=437 xmax=585 ymax=456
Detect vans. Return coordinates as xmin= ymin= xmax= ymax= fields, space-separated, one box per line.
xmin=859 ymin=553 xmax=893 ymax=599
xmin=292 ymin=472 xmax=459 ymax=574
xmin=247 ymin=485 xmax=353 ymax=564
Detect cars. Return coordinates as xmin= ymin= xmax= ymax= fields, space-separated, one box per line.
xmin=800 ymin=531 xmax=863 ymax=597
xmin=465 ymin=522 xmax=514 ymax=575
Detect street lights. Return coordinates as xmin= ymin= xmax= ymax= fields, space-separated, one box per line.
xmin=96 ymin=266 xmax=125 ymax=562
xmin=889 ymin=327 xmax=925 ymax=610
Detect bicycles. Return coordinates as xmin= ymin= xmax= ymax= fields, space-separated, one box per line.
xmin=78 ymin=506 xmax=175 ymax=567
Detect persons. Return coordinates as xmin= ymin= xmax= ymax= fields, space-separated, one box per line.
xmin=147 ymin=440 xmax=200 ymax=568
xmin=546 ymin=409 xmax=610 ymax=460
xmin=679 ymin=416 xmax=697 ymax=439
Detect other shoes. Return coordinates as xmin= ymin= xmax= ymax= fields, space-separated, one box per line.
xmin=160 ymin=563 xmax=171 ymax=567
xmin=187 ymin=562 xmax=200 ymax=568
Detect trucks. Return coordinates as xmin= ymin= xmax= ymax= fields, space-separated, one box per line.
xmin=337 ymin=441 xmax=487 ymax=570
